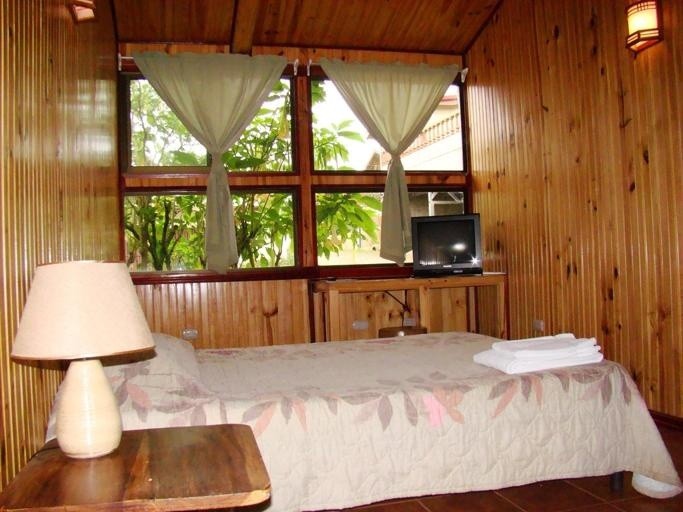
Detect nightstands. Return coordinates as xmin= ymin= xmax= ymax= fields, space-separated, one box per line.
xmin=0 ymin=424 xmax=272 ymax=512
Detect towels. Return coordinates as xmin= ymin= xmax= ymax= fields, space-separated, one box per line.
xmin=472 ymin=332 xmax=604 ymax=374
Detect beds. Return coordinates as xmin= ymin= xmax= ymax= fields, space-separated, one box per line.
xmin=43 ymin=330 xmax=634 ymax=511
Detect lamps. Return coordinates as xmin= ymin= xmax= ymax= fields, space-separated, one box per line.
xmin=10 ymin=260 xmax=157 ymax=460
xmin=624 ymin=0 xmax=664 ymax=54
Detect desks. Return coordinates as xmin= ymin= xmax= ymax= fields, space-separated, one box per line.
xmin=307 ymin=271 xmax=511 ymax=343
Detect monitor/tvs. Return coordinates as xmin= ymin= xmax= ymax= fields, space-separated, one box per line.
xmin=410 ymin=212 xmax=483 ymax=276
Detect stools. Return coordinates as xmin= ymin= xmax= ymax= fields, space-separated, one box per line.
xmin=378 ymin=327 xmax=428 ymax=338
